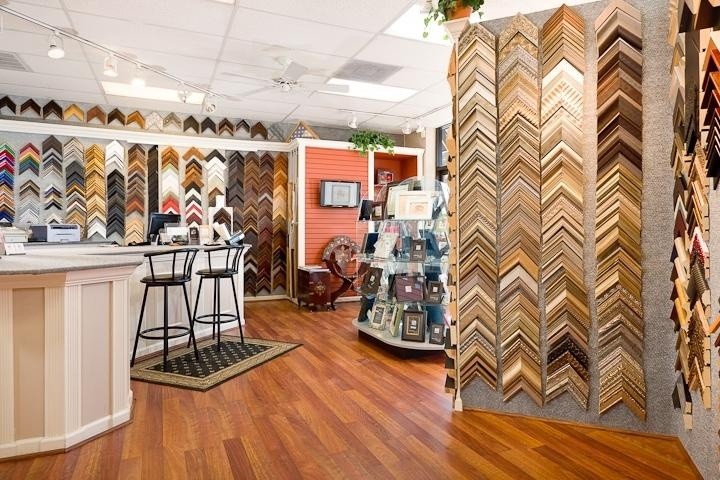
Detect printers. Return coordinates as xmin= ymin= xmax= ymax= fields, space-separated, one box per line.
xmin=28 ymin=224 xmax=80 ymax=243
xmin=0 ymin=222 xmax=28 ymax=255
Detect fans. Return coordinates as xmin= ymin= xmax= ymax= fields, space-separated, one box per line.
xmin=220 ymin=55 xmax=350 ymax=101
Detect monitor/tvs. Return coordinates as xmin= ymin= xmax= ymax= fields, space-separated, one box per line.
xmin=320 ymin=180 xmax=361 ymax=207
xmin=148 ymin=212 xmax=181 ymax=238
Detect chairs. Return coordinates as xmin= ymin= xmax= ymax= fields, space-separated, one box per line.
xmin=321 ymin=236 xmax=364 ymax=310
xmin=129 ymin=242 xmax=246 ymax=366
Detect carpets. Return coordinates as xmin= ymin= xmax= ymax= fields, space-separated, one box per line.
xmin=129 ymin=335 xmax=303 ymax=396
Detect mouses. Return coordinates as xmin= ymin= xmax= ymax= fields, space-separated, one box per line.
xmin=128 ymin=241 xmax=137 ymax=246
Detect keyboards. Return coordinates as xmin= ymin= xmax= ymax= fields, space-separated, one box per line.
xmin=137 ymin=242 xmax=150 ymax=245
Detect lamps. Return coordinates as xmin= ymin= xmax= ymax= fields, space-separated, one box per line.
xmin=47 ymin=30 xmax=120 ymax=78
xmin=347 ymin=112 xmax=412 ymax=137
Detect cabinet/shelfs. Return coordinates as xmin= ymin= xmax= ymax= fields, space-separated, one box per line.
xmin=354 ymin=175 xmax=452 ymax=359
xmin=298 ymin=265 xmax=331 ymax=311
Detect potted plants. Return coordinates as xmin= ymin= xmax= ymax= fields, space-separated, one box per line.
xmin=419 ymin=0 xmax=485 ymax=46
